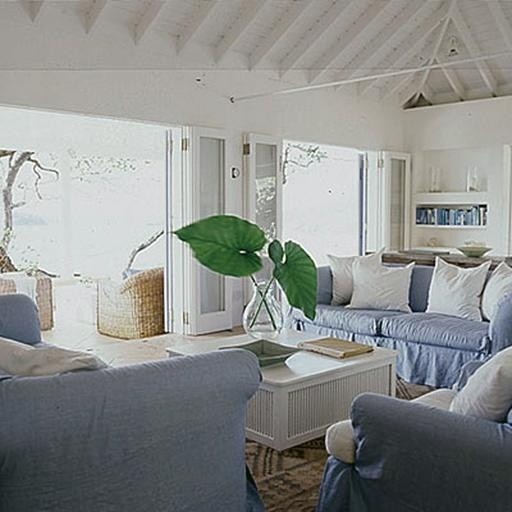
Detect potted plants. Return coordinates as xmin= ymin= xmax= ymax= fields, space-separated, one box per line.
xmin=171 ymin=215 xmax=317 ymax=339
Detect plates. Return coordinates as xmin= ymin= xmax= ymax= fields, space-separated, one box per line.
xmin=455 ymin=245 xmax=492 ymax=257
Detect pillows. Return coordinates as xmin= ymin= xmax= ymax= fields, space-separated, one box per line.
xmin=1 ymin=338 xmax=108 ymax=377
xmin=449 ymin=346 xmax=512 ymax=422
xmin=326 ymin=247 xmax=512 ymax=322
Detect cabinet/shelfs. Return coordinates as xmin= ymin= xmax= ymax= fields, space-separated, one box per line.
xmin=411 ymin=193 xmax=489 ymax=230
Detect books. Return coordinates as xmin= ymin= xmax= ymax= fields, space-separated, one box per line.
xmin=297 ymin=336 xmax=374 ymax=359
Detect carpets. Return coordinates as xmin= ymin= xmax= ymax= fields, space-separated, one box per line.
xmin=244 ymin=377 xmax=432 ymax=512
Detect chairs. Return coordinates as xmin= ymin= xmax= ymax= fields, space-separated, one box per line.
xmin=96 ymin=267 xmax=164 ymax=340
xmin=0 ymin=271 xmax=53 ymax=330
xmin=318 ymin=360 xmax=512 ymax=512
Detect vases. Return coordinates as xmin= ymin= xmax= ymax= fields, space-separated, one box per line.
xmin=467 ymin=166 xmax=481 ymax=192
xmin=429 ymin=167 xmax=443 ymax=192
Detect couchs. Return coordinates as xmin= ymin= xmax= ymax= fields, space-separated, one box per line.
xmin=0 ymin=294 xmax=264 ymax=512
xmin=280 ymin=263 xmax=512 ymax=389
